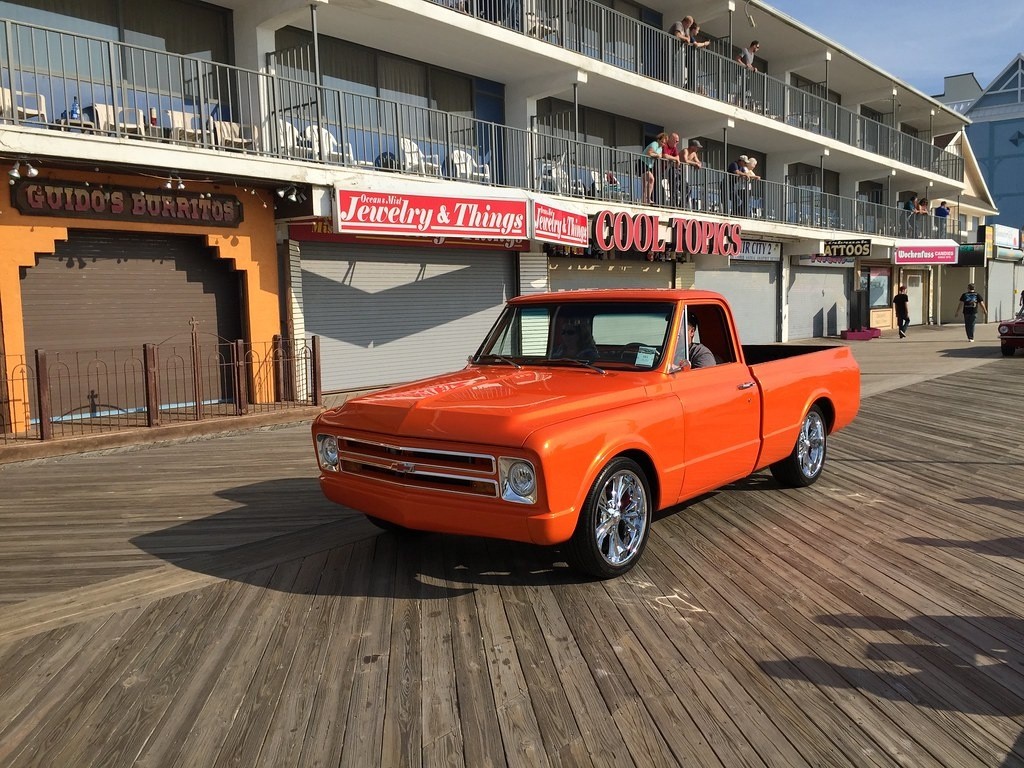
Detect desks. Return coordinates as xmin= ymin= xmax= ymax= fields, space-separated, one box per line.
xmin=51 ymin=118 xmax=96 ymax=135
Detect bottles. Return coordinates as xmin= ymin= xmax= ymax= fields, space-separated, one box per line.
xmin=71 ymin=96 xmax=80 ymax=119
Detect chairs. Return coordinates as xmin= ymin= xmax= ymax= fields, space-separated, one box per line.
xmin=1 ymin=90 xmax=693 ymax=208
xmin=528 ymin=17 xmax=562 ymax=45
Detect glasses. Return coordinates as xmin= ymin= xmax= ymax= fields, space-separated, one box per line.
xmin=756 ymin=47 xmax=759 ymax=49
xmin=743 ymin=161 xmax=747 ymax=164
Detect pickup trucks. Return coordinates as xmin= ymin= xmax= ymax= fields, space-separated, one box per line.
xmin=313 ymin=289 xmax=861 ymax=580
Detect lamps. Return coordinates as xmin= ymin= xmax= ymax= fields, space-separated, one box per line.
xmin=161 ymin=172 xmax=185 ymax=190
xmin=275 ymin=184 xmax=297 ymax=202
xmin=7 ymin=156 xmax=39 ymax=179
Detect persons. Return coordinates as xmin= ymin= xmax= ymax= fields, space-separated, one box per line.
xmin=666 ymin=312 xmax=716 ymax=368
xmin=736 ymin=40 xmax=760 ymax=110
xmin=1019 ymin=290 xmax=1024 ymax=316
xmin=893 ymin=286 xmax=910 ymax=338
xmin=661 ymin=16 xmax=710 ymax=93
xmin=635 ymin=132 xmax=703 ymax=211
xmin=721 ymin=155 xmax=760 ymax=218
xmin=954 ymin=283 xmax=987 ymax=343
xmin=552 ymin=317 xmax=600 ymax=360
xmin=935 ymin=201 xmax=950 ymax=239
xmin=900 ymin=196 xmax=928 ymax=239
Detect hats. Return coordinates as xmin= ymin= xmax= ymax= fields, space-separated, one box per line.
xmin=665 ymin=311 xmax=698 ymax=326
xmin=739 ymin=155 xmax=750 ymax=163
xmin=689 ymin=139 xmax=703 ymax=148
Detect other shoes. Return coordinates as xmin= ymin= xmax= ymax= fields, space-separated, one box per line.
xmin=900 ymin=335 xmax=903 ymax=338
xmin=969 ymin=339 xmax=974 ymax=342
xmin=650 ymin=201 xmax=654 ymax=203
xmin=900 ymin=330 xmax=906 ymax=337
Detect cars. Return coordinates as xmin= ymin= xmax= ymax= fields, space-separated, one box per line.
xmin=998 ymin=306 xmax=1024 ymax=356
